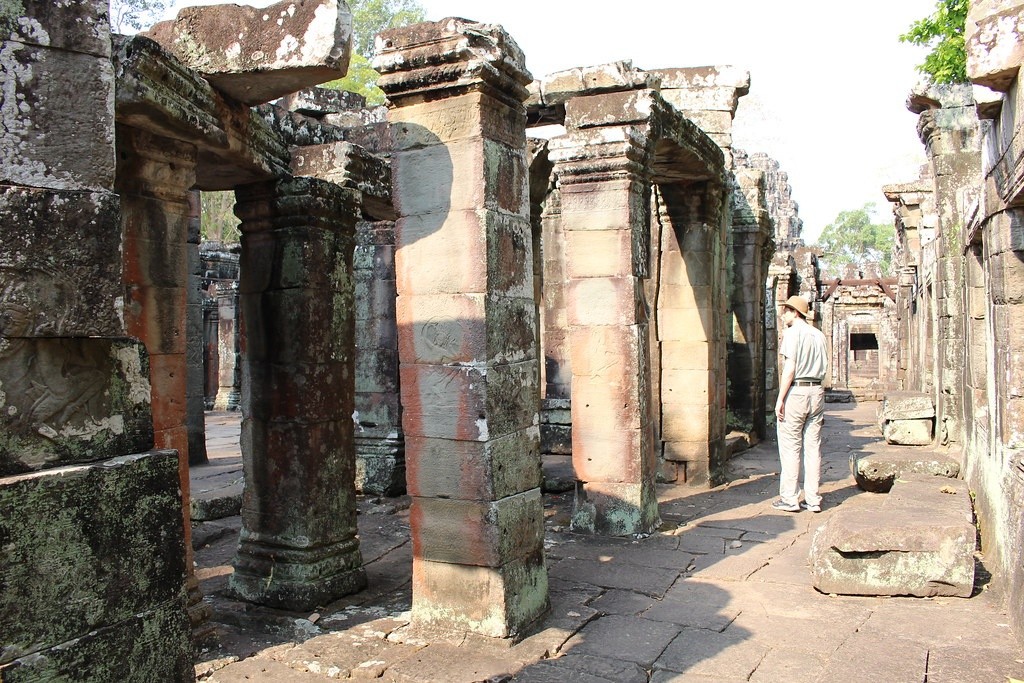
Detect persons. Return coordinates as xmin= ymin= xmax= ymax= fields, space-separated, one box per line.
xmin=772 ymin=295 xmax=827 ymax=511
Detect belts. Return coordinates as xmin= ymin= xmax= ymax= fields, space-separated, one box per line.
xmin=790 ymin=380 xmax=821 ymax=386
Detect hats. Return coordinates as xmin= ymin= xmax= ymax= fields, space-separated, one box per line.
xmin=777 ymin=295 xmax=814 ymax=319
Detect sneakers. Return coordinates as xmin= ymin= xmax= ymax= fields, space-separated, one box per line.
xmin=801 ymin=501 xmax=822 ymax=512
xmin=771 ymin=498 xmax=800 ymax=511
